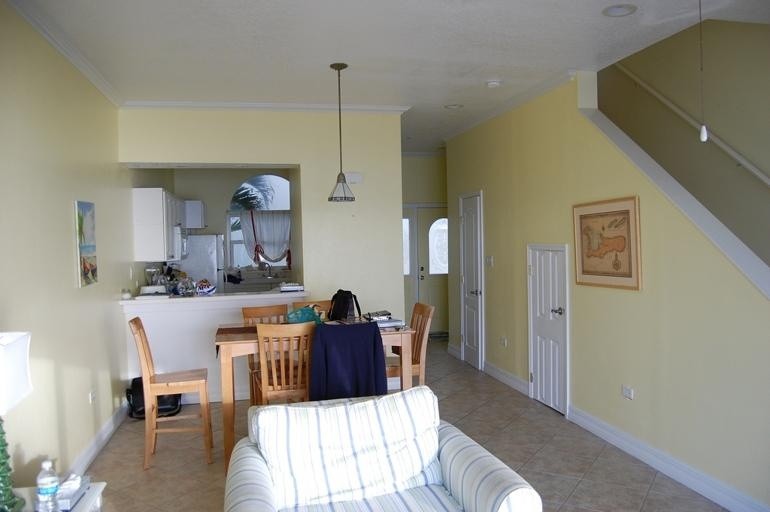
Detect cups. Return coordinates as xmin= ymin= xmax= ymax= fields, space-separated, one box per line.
xmin=121 ymin=288 xmax=132 ymax=300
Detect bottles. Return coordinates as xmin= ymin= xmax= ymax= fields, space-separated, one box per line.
xmin=34 ymin=460 xmax=60 ymax=512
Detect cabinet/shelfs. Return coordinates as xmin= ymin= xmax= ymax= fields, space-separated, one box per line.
xmin=130 ymin=187 xmax=204 ymax=263
xmin=223 ymin=281 xmax=287 ymax=293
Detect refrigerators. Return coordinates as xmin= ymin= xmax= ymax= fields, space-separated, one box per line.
xmin=177 ymin=233 xmax=227 ymax=294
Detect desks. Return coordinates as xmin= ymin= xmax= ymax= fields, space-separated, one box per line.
xmin=1 ymin=481 xmax=106 ymax=512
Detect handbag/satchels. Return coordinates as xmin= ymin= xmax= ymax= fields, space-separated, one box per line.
xmin=330 ymin=289 xmax=355 ymax=320
xmin=131 ymin=376 xmax=181 ymax=419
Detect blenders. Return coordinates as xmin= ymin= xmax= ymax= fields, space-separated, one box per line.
xmin=138 ymin=265 xmax=166 ymax=295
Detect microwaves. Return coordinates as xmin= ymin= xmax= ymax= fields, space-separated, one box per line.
xmin=174 ymin=226 xmax=189 ymax=261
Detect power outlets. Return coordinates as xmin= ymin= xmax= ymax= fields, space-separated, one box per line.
xmin=90 ymin=389 xmax=97 ymax=402
xmin=621 ymin=385 xmax=633 ymax=399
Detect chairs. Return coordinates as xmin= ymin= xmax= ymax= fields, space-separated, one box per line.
xmin=223 ymin=385 xmax=542 ymax=511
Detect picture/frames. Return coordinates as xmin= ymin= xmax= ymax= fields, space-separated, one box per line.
xmin=572 ymin=195 xmax=641 ymax=291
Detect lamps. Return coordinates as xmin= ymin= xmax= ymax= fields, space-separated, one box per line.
xmin=328 ymin=64 xmax=355 ymax=201
xmin=699 ymin=1 xmax=707 ymax=141
xmin=0 ymin=331 xmax=33 ymax=511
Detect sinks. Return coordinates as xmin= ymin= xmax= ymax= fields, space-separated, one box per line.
xmin=263 ymin=276 xmax=279 ymax=279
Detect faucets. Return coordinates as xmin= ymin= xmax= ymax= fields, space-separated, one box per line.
xmin=265 ymin=263 xmax=271 ymax=274
